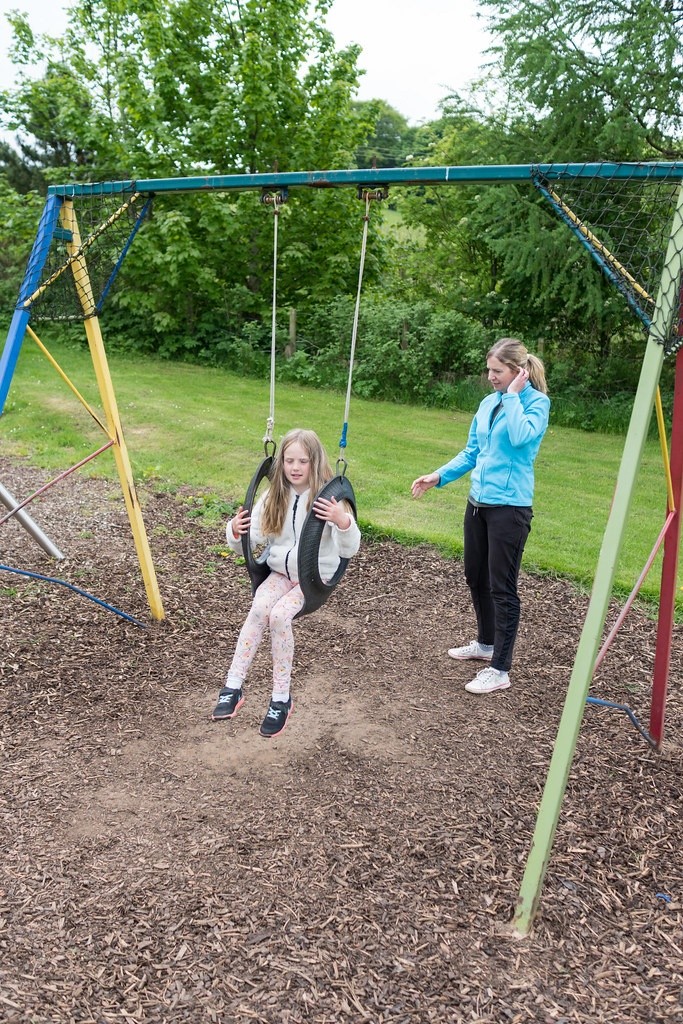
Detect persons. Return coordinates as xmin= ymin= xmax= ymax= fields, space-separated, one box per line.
xmin=212 ymin=428 xmax=362 ymax=737
xmin=411 ymin=338 xmax=551 ymax=694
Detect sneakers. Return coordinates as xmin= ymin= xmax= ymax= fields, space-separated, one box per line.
xmin=259 ymin=694 xmax=293 ymax=737
xmin=211 ymin=686 xmax=244 ymax=719
xmin=465 ymin=667 xmax=511 ymax=694
xmin=447 ymin=640 xmax=494 ymax=660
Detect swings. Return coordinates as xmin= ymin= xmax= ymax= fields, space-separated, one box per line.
xmin=240 ymin=184 xmax=378 ymax=616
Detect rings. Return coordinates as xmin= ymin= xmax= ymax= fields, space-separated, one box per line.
xmin=525 ymin=375 xmax=527 ymax=379
